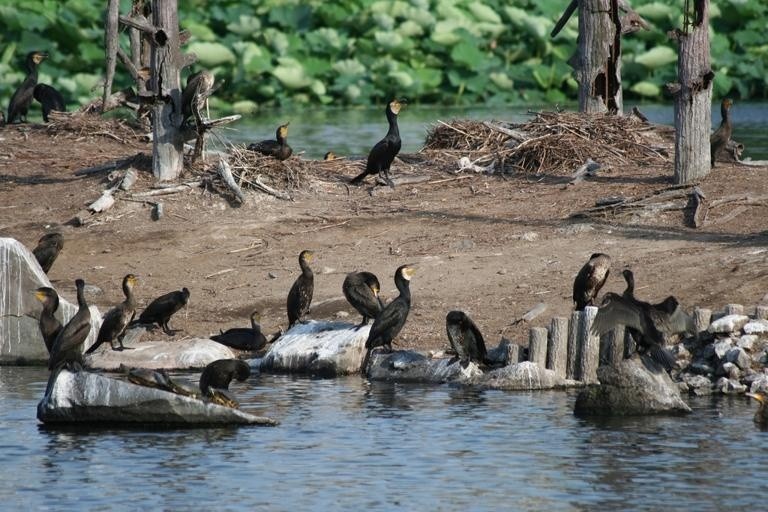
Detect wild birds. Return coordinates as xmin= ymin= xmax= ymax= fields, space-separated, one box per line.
xmin=446 ymin=310 xmax=488 ymax=363
xmin=31 ymin=231 xmax=67 ymax=274
xmin=208 ymin=311 xmax=267 ymax=353
xmin=6 ymin=49 xmax=65 ymax=127
xmin=574 ymin=251 xmax=696 ymax=370
xmin=710 ymin=96 xmax=736 ymax=170
xmin=246 ymin=122 xmax=293 ymax=161
xmin=199 ymin=357 xmax=252 ymax=405
xmin=343 ymin=262 xmax=422 ymax=353
xmin=27 ymin=272 xmax=191 ymax=371
xmin=744 ymin=390 xmax=768 ymax=425
xmin=350 ymin=98 xmax=411 ymax=189
xmin=284 ymin=249 xmax=316 ymax=329
